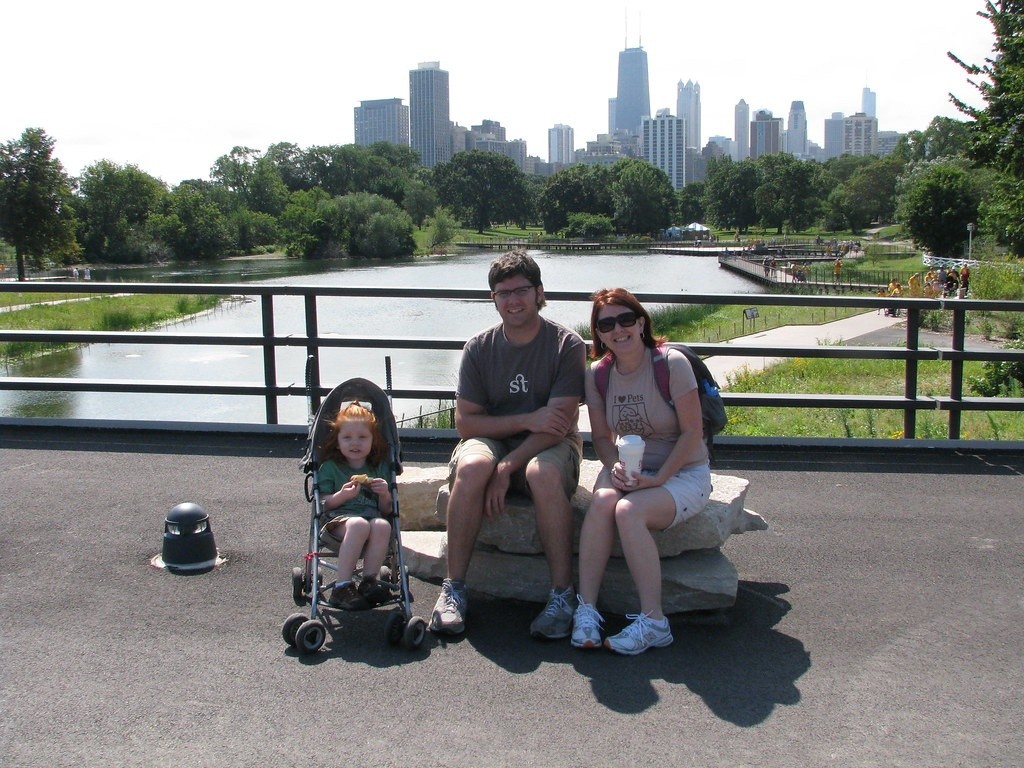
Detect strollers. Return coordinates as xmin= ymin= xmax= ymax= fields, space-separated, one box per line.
xmin=884 ymin=293 xmax=898 ymax=317
xmin=797 ymin=269 xmax=805 ymax=283
xmin=282 ymin=377 xmax=428 ymax=658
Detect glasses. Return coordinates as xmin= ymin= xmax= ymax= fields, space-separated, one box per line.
xmin=596 ymin=312 xmax=641 ymax=333
xmin=493 ymin=286 xmax=535 ymax=297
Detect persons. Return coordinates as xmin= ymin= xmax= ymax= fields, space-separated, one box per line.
xmin=875 ymin=262 xmax=970 ymax=317
xmin=73 ymin=268 xmax=79 ymax=280
xmin=428 ymin=248 xmax=587 ymax=641
xmin=568 ymin=287 xmax=711 ymax=656
xmin=0 ymin=263 xmax=6 ymax=281
xmin=709 ymin=229 xmax=860 ymax=282
xmin=83 ymin=267 xmax=91 ymax=280
xmin=314 ymin=399 xmax=394 ymax=611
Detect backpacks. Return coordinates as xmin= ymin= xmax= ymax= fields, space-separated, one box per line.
xmin=595 ymin=344 xmax=727 ymax=438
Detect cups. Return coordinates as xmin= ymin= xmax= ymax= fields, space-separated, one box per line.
xmin=618 ymin=435 xmax=646 ymax=480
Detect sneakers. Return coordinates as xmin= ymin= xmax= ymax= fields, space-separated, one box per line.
xmin=604 ymin=613 xmax=673 ymax=655
xmin=530 ymin=586 xmax=576 ymax=639
xmin=329 ymin=582 xmax=366 ymax=611
xmin=429 ymin=579 xmax=468 ymax=634
xmin=358 ymin=577 xmax=388 ymax=603
xmin=572 ymin=604 xmax=602 ymax=648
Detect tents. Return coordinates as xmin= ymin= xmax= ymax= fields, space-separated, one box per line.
xmin=665 ymin=226 xmax=681 ymax=234
xmin=685 ymin=222 xmax=710 ymax=232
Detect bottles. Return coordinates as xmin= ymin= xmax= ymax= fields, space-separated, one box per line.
xmin=702 ymin=376 xmax=727 ymax=422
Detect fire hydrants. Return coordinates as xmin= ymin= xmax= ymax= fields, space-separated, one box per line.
xmin=832 ymin=257 xmax=841 ymax=280
